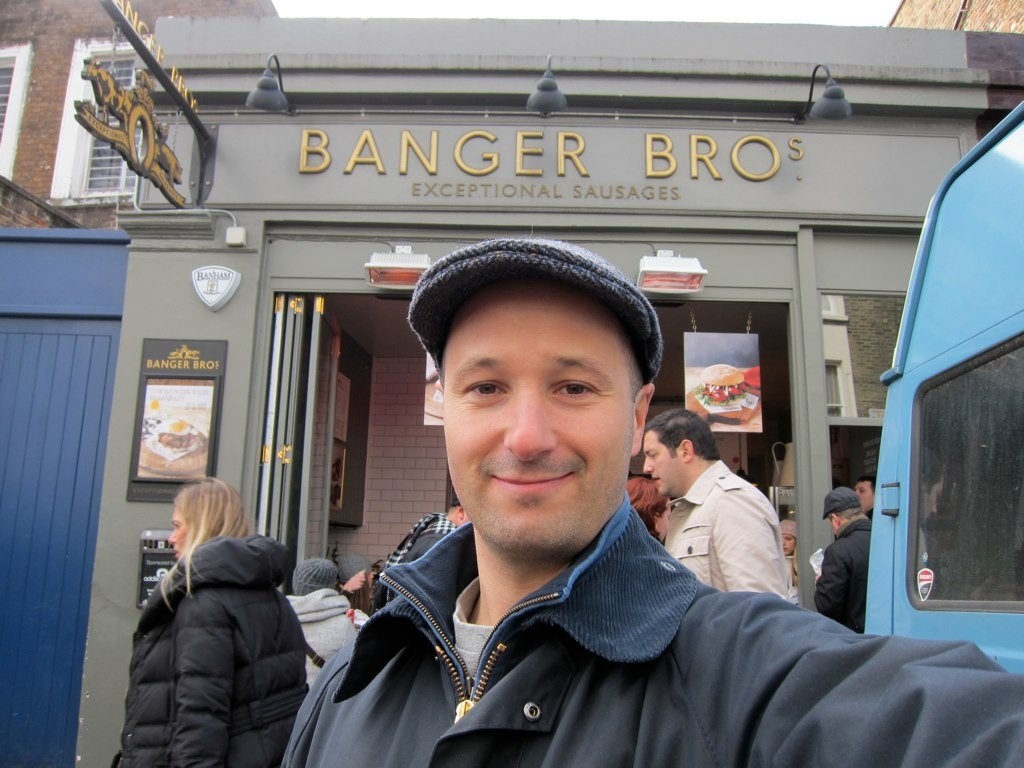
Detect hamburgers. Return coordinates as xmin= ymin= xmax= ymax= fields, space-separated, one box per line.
xmin=694 ymin=363 xmax=748 ymax=407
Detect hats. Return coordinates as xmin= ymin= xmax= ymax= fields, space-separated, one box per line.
xmin=292 ymin=558 xmax=337 ymax=596
xmin=780 ymin=519 xmax=796 ymax=539
xmin=407 ymin=238 xmax=664 ymax=385
xmin=822 ymin=486 xmax=862 ymax=520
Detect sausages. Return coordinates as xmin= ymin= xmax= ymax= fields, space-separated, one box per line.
xmin=157 ymin=433 xmax=196 ymax=448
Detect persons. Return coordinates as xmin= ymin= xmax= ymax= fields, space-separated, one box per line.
xmin=110 ymin=477 xmax=309 ymax=768
xmin=281 ymin=239 xmax=1023 ymax=768
xmin=642 ymin=409 xmax=790 ymax=603
xmin=286 ymin=471 xmax=886 ymax=684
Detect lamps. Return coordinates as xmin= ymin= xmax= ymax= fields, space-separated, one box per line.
xmin=363 ymin=246 xmax=432 ymax=290
xmin=245 ymin=53 xmax=296 ymax=116
xmin=524 ymin=54 xmax=567 ymax=118
xmin=635 ymin=248 xmax=707 ymax=294
xmin=796 ymin=64 xmax=851 ymax=125
xmin=779 ymin=441 xmax=794 ymax=488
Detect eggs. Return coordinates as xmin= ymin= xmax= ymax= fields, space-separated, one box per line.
xmin=154 ymin=417 xmax=192 ymax=435
